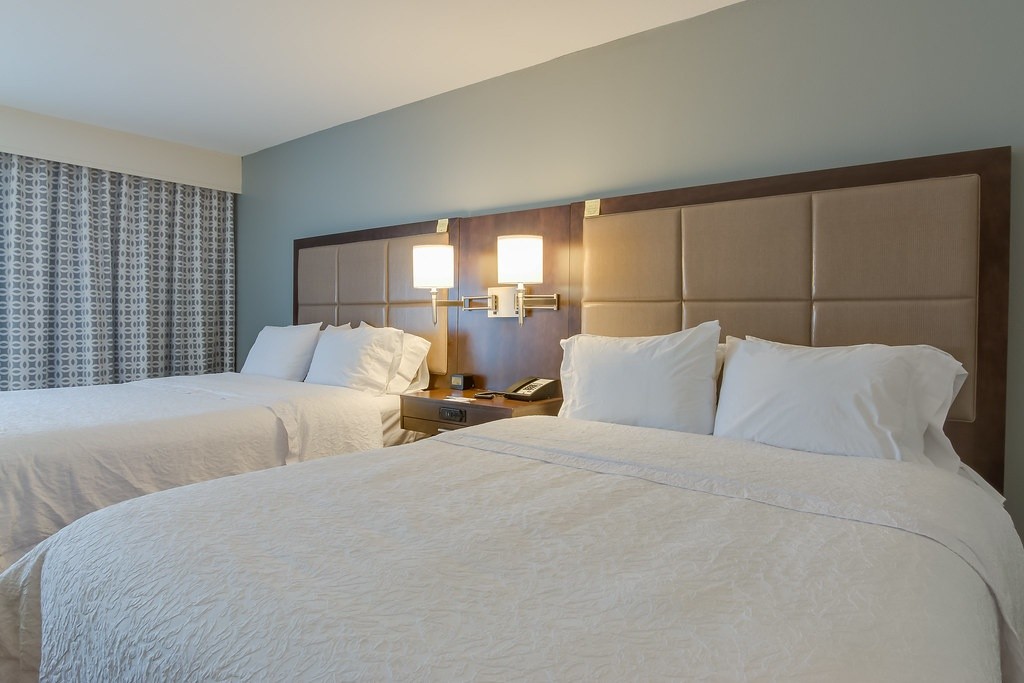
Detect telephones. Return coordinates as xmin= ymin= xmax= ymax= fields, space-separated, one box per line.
xmin=503 ymin=376 xmax=557 ymax=402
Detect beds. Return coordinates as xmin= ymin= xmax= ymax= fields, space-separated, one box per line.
xmin=0 ymin=415 xmax=1024 ymax=682
xmin=1 ymin=370 xmax=437 ymax=572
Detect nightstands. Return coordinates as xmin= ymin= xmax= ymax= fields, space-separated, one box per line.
xmin=399 ymin=388 xmax=562 ymax=436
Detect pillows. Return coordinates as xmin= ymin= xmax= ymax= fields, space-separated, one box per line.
xmin=319 ymin=321 xmax=352 ymax=340
xmin=239 ymin=319 xmax=324 ymax=382
xmin=358 ymin=320 xmax=432 ymax=392
xmin=304 ymin=324 xmax=406 ymax=391
xmin=560 ymin=320 xmax=967 ymax=459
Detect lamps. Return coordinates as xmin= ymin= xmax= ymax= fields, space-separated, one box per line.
xmin=413 ymin=243 xmax=471 ymax=326
xmin=497 ymin=231 xmax=557 ymax=324
